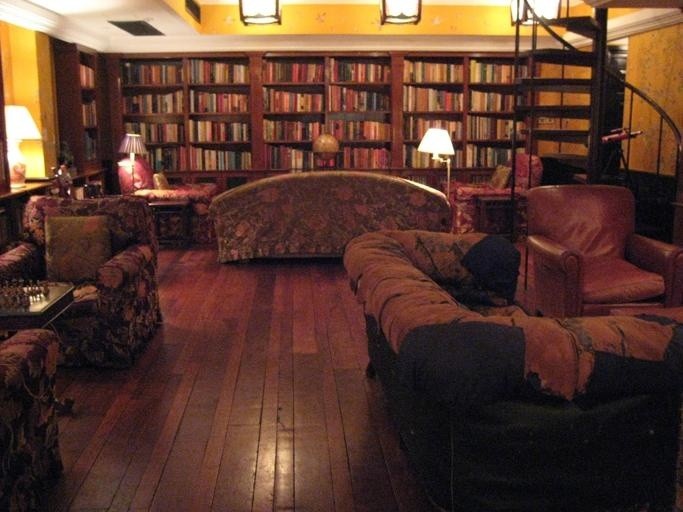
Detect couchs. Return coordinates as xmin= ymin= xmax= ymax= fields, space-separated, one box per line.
xmin=337 ymin=229 xmax=683 ymax=512
xmin=209 ymin=168 xmax=452 ymax=261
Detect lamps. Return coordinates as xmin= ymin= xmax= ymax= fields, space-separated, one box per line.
xmin=507 ymin=0 xmax=562 ymax=27
xmin=376 ymin=0 xmax=422 ymax=27
xmin=3 ymin=104 xmax=43 ymax=189
xmin=236 ymin=0 xmax=283 ymax=27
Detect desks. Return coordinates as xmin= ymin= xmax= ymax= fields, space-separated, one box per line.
xmin=0 ymin=282 xmax=76 ymax=413
xmin=606 ymin=305 xmax=683 ymax=326
xmin=474 ymin=192 xmax=519 ymax=241
xmin=148 ymin=200 xmax=191 ymax=247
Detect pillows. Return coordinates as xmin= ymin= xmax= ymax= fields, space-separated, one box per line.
xmin=41 ymin=215 xmax=113 ymax=283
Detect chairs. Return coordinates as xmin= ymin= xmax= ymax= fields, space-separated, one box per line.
xmin=524 ymin=183 xmax=683 ymax=316
xmin=116 ymin=156 xmax=218 ymax=244
xmin=440 ymin=153 xmax=543 ymax=236
xmin=0 ymin=194 xmax=165 ymax=381
xmin=0 ymin=327 xmax=65 ymax=510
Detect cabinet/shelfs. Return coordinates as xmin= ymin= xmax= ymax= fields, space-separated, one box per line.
xmin=186 ymin=54 xmax=256 ymax=176
xmin=104 ymin=54 xmax=186 ymax=177
xmin=256 ymin=54 xmax=328 ymax=172
xmin=468 ymin=54 xmax=538 ymax=171
xmin=55 ymin=42 xmax=105 ymax=186
xmin=328 ymin=54 xmax=397 ymax=170
xmin=398 ymin=55 xmax=467 ymax=170
